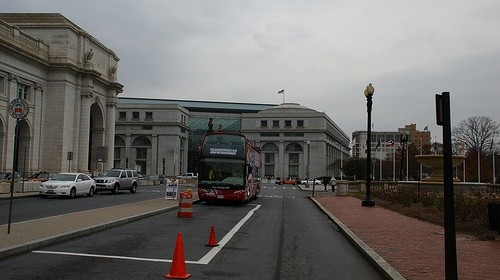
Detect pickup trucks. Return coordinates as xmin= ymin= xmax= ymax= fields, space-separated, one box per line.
xmin=182 ymin=172 xmax=197 ymax=178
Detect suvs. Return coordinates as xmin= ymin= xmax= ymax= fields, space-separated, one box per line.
xmin=92 ymin=169 xmax=138 ymax=195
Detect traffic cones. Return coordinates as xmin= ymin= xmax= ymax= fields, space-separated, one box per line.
xmin=205 ymin=225 xmax=220 ymax=247
xmin=164 ymin=231 xmax=194 ymax=278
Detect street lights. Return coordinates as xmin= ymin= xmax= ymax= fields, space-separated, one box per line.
xmin=305 ymin=139 xmax=311 ymax=187
xmin=361 ymin=82 xmax=376 ymax=206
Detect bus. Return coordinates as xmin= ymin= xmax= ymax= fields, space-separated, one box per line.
xmin=197 ymin=131 xmax=262 ymax=204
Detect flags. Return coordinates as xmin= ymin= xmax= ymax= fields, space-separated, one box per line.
xmin=373 ymin=138 xmax=380 ymax=151
xmin=385 ymin=137 xmax=394 ymax=147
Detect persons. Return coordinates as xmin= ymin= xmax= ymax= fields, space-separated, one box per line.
xmin=330 ymin=176 xmax=337 ymax=192
xmin=323 ymin=177 xmax=328 ymax=190
xmin=209 ymin=167 xmax=222 ymax=179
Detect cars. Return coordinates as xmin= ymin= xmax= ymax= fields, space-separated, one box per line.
xmin=40 ymin=173 xmax=97 ymax=198
xmin=0 ymin=170 xmax=28 ymax=183
xmin=301 ymin=178 xmax=321 ymax=185
xmin=29 ymin=171 xmax=59 ymax=182
xmin=275 ymin=176 xmax=298 ymax=185
